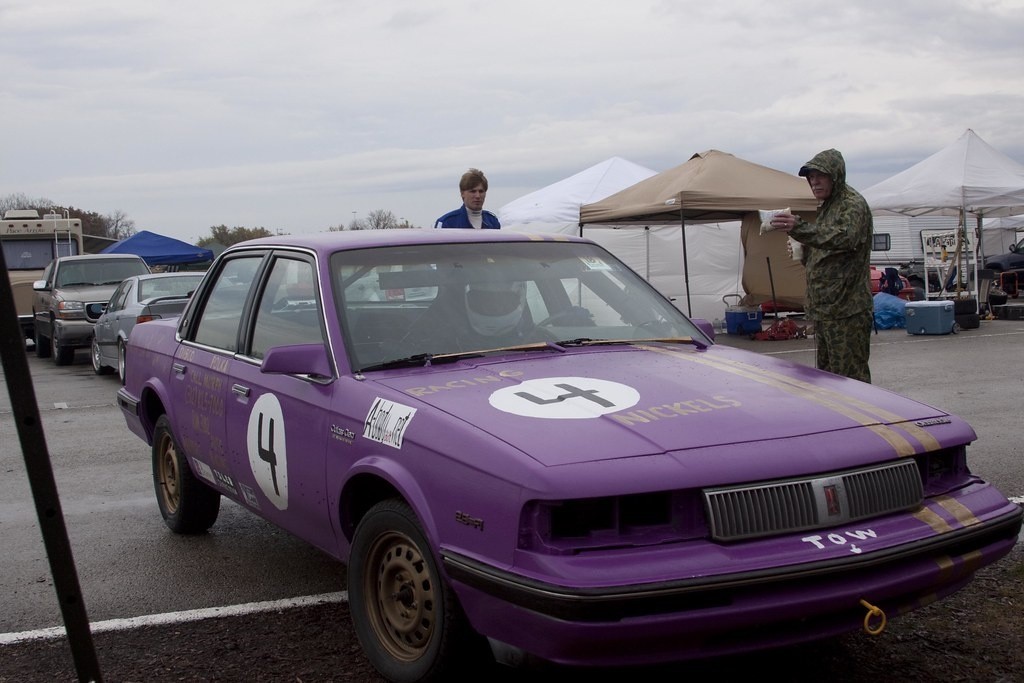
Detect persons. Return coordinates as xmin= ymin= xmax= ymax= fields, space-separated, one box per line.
xmin=434 ymin=168 xmax=499 ymax=232
xmin=770 ymin=148 xmax=876 ymax=386
xmin=436 ymin=256 xmax=564 ymax=358
xmin=882 ymin=268 xmax=905 ymax=297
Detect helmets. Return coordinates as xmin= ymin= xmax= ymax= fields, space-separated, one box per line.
xmin=462 ymin=281 xmax=526 ymax=338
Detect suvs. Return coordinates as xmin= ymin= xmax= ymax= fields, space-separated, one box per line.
xmin=0 ymin=206 xmax=85 ymax=353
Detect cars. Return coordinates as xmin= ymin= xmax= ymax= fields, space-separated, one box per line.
xmin=868 ymin=263 xmax=911 ymax=303
xmin=90 ymin=272 xmax=244 ymax=387
xmin=984 ymin=237 xmax=1024 ymax=299
xmin=30 ymin=252 xmax=157 ymax=372
xmin=112 ymin=224 xmax=1023 ymax=683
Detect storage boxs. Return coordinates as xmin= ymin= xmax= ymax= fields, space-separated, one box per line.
xmin=724 ymin=304 xmax=763 ymax=335
xmin=905 ymin=301 xmax=961 ymax=336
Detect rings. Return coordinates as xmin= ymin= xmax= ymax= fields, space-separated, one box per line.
xmin=785 ymin=224 xmax=788 ymax=229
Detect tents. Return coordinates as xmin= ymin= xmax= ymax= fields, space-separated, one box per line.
xmin=979 ymin=215 xmax=1024 ymax=260
xmin=99 ymin=232 xmax=216 ymax=275
xmin=577 ymin=149 xmax=828 ymax=335
xmin=860 ymin=128 xmax=1024 ymax=317
xmin=499 ymin=157 xmax=764 ymax=333
